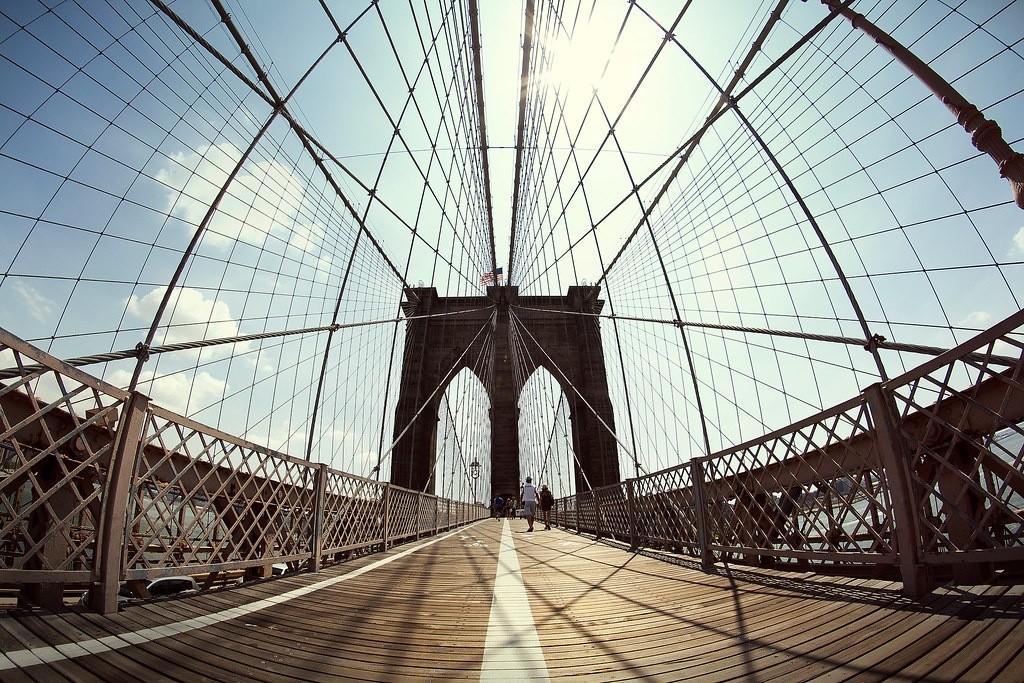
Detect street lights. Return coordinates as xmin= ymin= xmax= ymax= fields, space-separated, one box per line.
xmin=469 ymin=457 xmax=480 ymax=502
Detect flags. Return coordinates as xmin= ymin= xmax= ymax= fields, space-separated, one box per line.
xmin=480 ymin=267 xmax=502 ymax=286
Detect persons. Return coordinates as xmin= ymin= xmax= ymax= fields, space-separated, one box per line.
xmin=506 ymin=498 xmax=511 ymax=520
xmin=521 ymin=476 xmax=540 ymax=532
xmin=494 ymin=494 xmax=505 ymax=522
xmin=509 ymin=496 xmax=517 ymax=520
xmin=540 ymin=484 xmax=555 ymax=530
